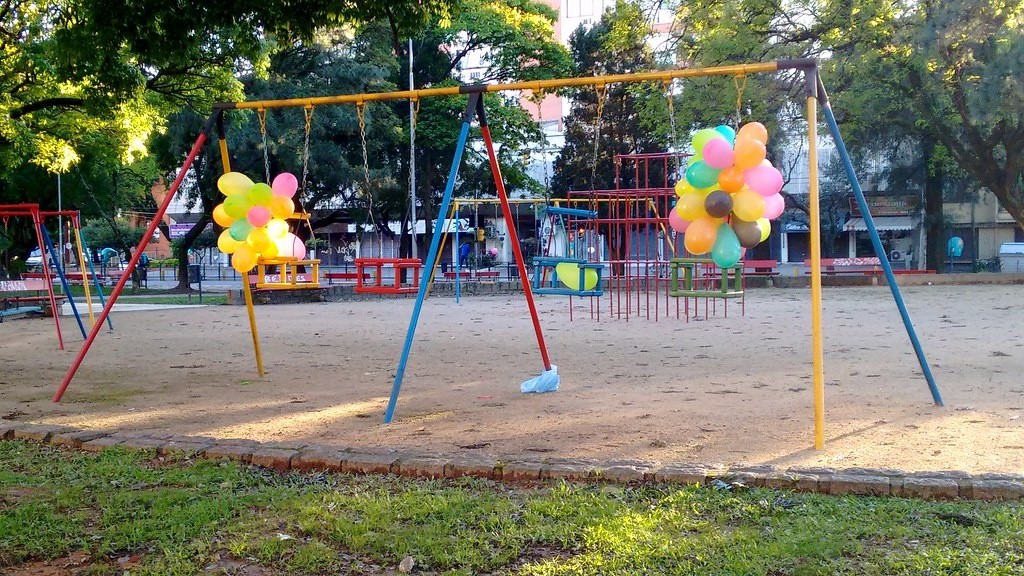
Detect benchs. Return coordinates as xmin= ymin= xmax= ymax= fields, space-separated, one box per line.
xmin=444 ymin=272 xmax=500 ymax=284
xmin=803 ymin=257 xmax=887 ymax=286
xmin=324 ymin=270 xmax=372 ymax=285
xmin=64 ymin=273 xmax=101 ymax=286
xmin=0 ymin=294 xmax=68 ymax=317
xmin=699 ymin=259 xmax=781 ymax=289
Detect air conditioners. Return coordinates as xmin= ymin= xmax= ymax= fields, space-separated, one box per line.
xmin=891 ymin=249 xmax=905 ymax=261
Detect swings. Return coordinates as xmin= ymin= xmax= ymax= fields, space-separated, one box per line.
xmin=256 ymin=105 xmax=322 ymax=291
xmin=531 ymin=84 xmax=606 ymax=297
xmin=353 ymin=97 xmax=422 ymax=294
xmin=661 ymin=73 xmax=746 ymax=298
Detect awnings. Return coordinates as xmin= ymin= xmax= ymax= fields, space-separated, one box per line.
xmin=842 ymin=217 xmax=914 ymax=232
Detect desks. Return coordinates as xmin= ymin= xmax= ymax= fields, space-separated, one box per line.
xmin=20 ymin=272 xmax=56 ymax=286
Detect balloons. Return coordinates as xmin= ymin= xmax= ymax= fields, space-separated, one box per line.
xmin=213 ymin=172 xmax=306 ymax=273
xmin=555 ymin=262 xmax=598 ymax=291
xmin=668 ymin=122 xmax=784 ymax=269
xmin=491 ymin=248 xmax=498 ymax=254
